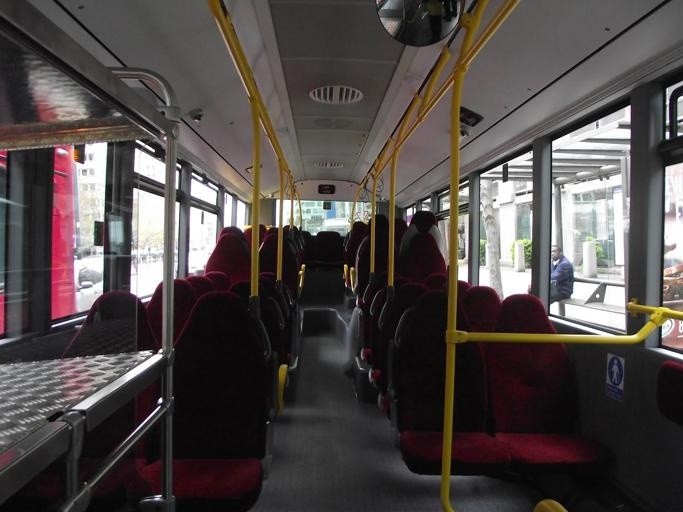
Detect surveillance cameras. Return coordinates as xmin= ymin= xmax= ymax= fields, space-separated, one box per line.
xmin=190 ymin=109 xmax=206 ymax=123
xmin=459 ymin=125 xmax=469 ymax=137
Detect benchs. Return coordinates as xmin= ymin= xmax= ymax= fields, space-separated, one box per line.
xmin=557 ymin=277 xmax=625 ymax=316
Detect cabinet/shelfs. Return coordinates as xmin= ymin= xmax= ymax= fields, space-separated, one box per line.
xmin=0 ymin=0 xmax=181 ymax=512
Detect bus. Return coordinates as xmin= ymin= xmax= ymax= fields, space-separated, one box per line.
xmin=320 ymin=216 xmax=359 ymax=236
xmin=0 ymin=143 xmax=85 ymax=335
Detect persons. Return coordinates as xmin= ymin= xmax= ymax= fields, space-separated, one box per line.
xmin=528 ymin=245 xmax=574 ymax=305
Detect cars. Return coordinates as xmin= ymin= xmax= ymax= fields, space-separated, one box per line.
xmin=73 ymin=254 xmax=103 ymax=313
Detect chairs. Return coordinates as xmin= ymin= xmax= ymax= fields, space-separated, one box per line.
xmin=13 ymin=210 xmax=605 ymax=512
